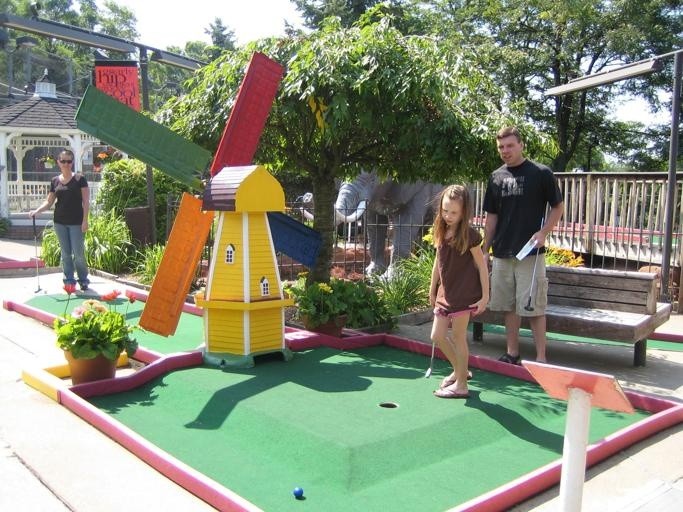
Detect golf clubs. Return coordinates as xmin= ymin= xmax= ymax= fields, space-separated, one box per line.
xmin=32 ymin=216 xmax=42 ymax=293
xmin=424 ymin=343 xmax=436 ymax=377
xmin=525 ymin=218 xmax=544 ymax=312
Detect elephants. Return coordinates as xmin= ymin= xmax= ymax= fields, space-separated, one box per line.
xmin=298 ymin=162 xmax=475 ymax=282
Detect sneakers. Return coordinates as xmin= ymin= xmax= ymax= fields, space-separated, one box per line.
xmin=80 ymin=282 xmax=88 ymax=289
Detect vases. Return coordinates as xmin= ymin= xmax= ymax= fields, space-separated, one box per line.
xmin=301 ymin=314 xmax=347 ymax=337
xmin=64 ymin=350 xmax=116 ymax=385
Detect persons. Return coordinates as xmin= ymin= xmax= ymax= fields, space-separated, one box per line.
xmin=427 ymin=184 xmax=490 ymax=397
xmin=481 ymin=126 xmax=564 ymax=367
xmin=27 ymin=149 xmax=90 ymax=291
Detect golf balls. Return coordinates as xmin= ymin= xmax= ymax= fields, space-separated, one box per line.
xmin=293 ymin=487 xmax=303 ymax=498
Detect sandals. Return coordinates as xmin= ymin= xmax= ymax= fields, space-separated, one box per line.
xmin=498 ymin=353 xmax=520 ymax=363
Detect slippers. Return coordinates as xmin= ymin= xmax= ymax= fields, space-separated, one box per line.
xmin=433 ymin=370 xmax=473 ymax=397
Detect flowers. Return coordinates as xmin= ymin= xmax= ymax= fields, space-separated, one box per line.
xmin=53 ymin=283 xmax=149 ymax=359
xmin=283 ymin=270 xmax=348 ymax=327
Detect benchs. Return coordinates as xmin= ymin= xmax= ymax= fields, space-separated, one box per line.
xmin=472 ymin=266 xmax=672 ymax=367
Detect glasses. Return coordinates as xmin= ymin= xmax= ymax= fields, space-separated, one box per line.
xmin=60 ymin=160 xmax=73 ymax=163
